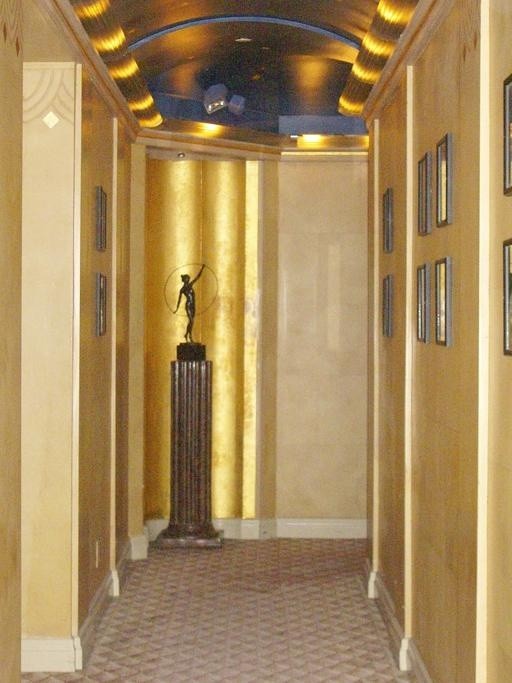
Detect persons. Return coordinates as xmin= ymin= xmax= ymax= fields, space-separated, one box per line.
xmin=172 ymin=262 xmax=206 ymax=341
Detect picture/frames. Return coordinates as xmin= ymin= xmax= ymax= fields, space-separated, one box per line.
xmin=94 ymin=185 xmax=108 ymax=337
xmin=501 ymin=75 xmax=512 ymax=355
xmin=416 ymin=133 xmax=451 ymax=348
xmin=382 ymin=187 xmax=393 ymax=339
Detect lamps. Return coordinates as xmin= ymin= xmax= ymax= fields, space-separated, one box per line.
xmin=201 ymin=82 xmax=248 ymax=118
